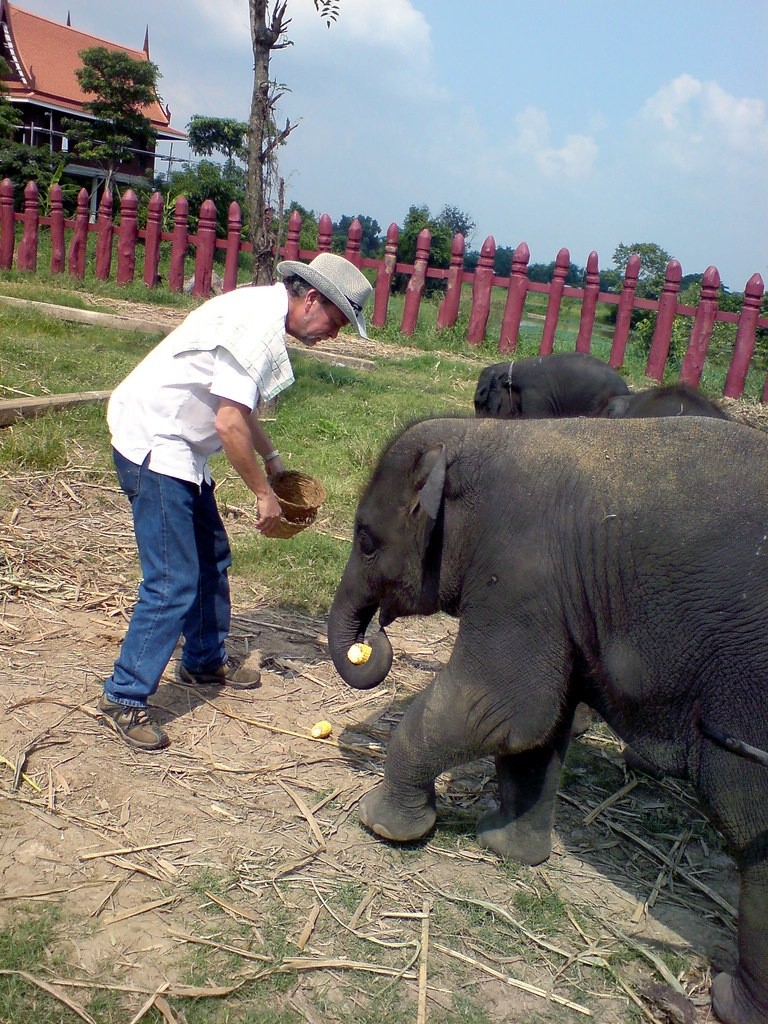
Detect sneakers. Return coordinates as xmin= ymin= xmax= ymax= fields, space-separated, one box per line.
xmin=180 ymin=656 xmax=261 ymax=690
xmin=96 ymin=690 xmax=171 ymax=750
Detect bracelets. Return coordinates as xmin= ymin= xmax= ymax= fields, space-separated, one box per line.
xmin=262 ymin=449 xmax=280 ymax=464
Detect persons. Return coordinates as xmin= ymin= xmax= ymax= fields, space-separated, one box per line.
xmin=98 ymin=251 xmax=376 ymax=752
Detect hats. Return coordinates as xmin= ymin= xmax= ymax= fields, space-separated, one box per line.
xmin=277 ymin=253 xmax=372 ymax=341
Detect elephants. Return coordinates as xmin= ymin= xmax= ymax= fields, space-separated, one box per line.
xmin=327 ymin=353 xmax=768 ymax=1024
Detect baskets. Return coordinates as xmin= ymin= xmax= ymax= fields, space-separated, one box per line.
xmin=257 ymin=470 xmax=326 ymax=539
